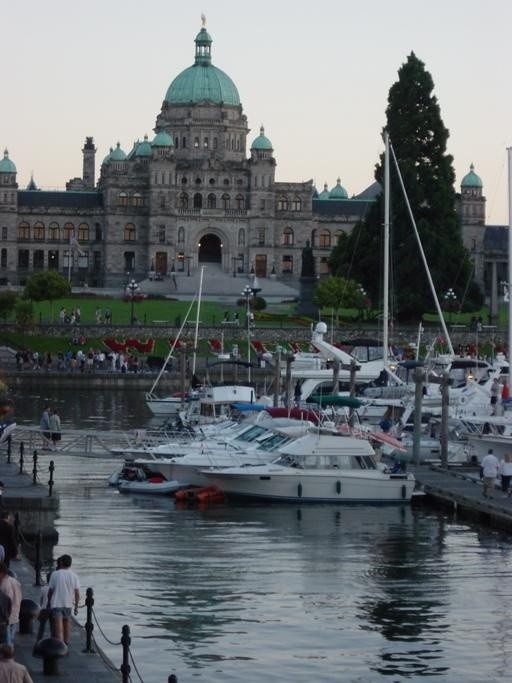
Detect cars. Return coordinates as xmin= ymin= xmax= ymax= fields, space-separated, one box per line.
xmin=0 ymin=346 xmax=26 ymax=366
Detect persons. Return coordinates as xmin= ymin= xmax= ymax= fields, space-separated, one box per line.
xmin=222 ymin=308 xmax=231 ymax=328
xmin=498 ymin=452 xmax=512 ymax=494
xmin=14 ymin=346 xmax=140 ymax=373
xmin=248 ymin=312 xmax=250 ymax=318
xmin=245 ymin=310 xmax=251 ymax=328
xmin=59 ymin=303 xmax=114 ymax=325
xmin=50 ymin=410 xmax=63 ymax=450
xmin=36 ymin=573 xmax=61 ymax=641
xmin=499 ymin=380 xmax=512 ymax=418
xmin=232 ymin=309 xmax=240 ymax=327
xmin=0 ymin=510 xmax=34 ymax=683
xmin=40 ymin=405 xmax=51 ymax=451
xmin=45 ymin=555 xmax=81 ymax=645
xmin=479 ymin=448 xmax=501 ymax=500
xmin=488 ymin=376 xmax=498 ymax=417
